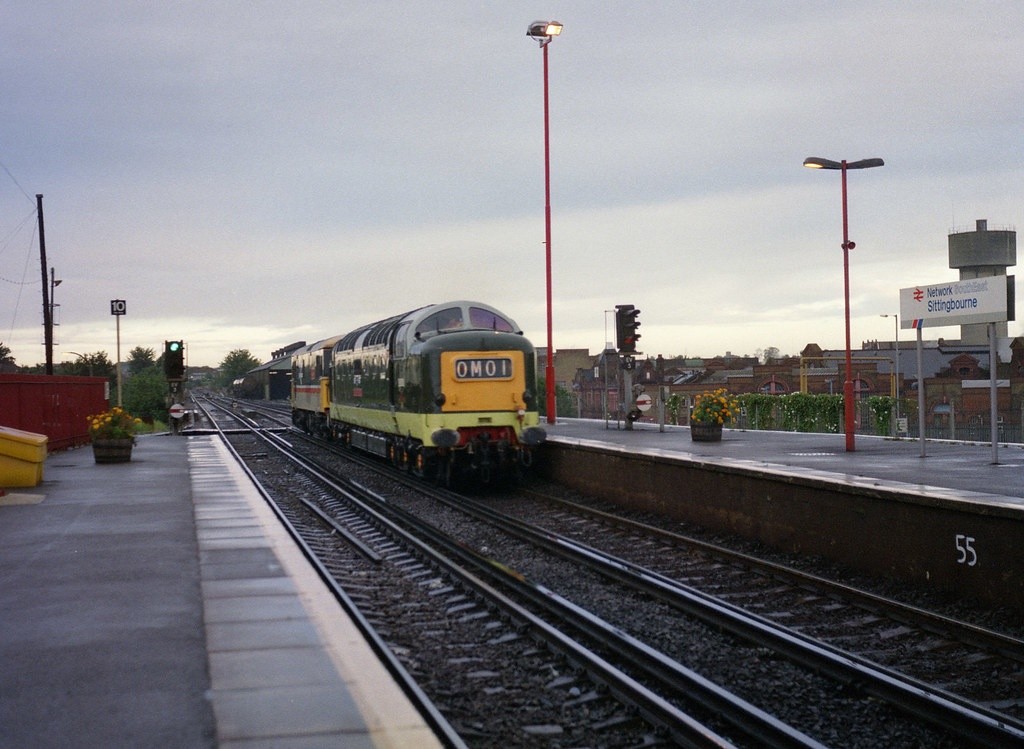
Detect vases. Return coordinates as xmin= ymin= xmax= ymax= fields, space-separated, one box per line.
xmin=90 ymin=439 xmax=134 ymax=465
xmin=689 ymin=420 xmax=723 ymax=442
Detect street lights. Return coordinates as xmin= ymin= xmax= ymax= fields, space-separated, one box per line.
xmin=526 ymin=21 xmax=563 ymax=423
xmin=804 ymin=157 xmax=884 ymax=452
xmin=880 ymin=314 xmax=899 ymax=437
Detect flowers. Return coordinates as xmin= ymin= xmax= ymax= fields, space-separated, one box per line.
xmin=690 ymin=389 xmax=740 ymax=424
xmin=86 ymin=407 xmax=148 ymax=438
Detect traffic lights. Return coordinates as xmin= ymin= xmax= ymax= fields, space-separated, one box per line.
xmin=615 ymin=305 xmax=641 ymax=351
xmin=164 ymin=339 xmax=185 ymax=377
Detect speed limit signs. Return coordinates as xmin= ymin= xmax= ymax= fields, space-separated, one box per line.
xmin=111 ymin=300 xmax=126 ymax=315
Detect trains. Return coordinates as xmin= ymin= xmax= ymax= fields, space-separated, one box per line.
xmin=290 ymin=300 xmax=547 ymax=487
